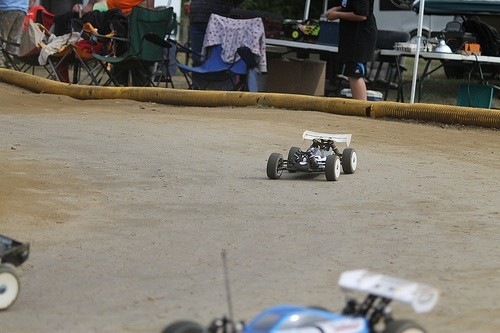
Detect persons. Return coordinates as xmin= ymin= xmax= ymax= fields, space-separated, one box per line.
xmin=324 ymin=0 xmax=378 ymax=101
xmin=189 ymin=0 xmax=232 ymax=84
xmin=0 ymin=0 xmax=155 ymax=88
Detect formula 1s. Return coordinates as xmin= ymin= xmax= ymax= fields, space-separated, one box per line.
xmin=161 ymin=269 xmax=438 ymax=332
xmin=266 ymin=130 xmax=357 ymax=181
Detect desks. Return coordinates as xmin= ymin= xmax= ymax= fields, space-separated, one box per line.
xmin=380 ymin=50 xmax=500 ymax=110
xmin=265 ymin=38 xmax=339 ymax=52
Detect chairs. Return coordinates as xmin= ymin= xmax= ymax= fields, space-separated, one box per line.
xmin=318 ymin=21 xmax=411 ymax=89
xmin=0 ymin=6 xmax=267 ymax=92
